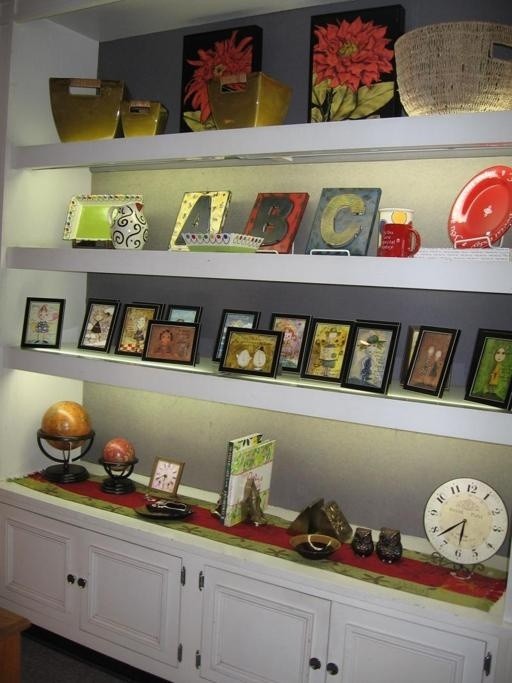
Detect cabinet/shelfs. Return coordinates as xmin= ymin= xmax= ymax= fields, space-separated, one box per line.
xmin=0 ymin=0 xmax=512 ymax=622
xmin=0 ymin=502 xmax=190 ymax=682
xmin=190 ymin=550 xmax=510 ymax=682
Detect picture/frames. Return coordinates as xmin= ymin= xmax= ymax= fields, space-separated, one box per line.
xmin=21 ymin=297 xmax=64 ymax=349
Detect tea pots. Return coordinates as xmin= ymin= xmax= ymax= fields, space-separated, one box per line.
xmin=106 ymin=201 xmax=150 ymax=250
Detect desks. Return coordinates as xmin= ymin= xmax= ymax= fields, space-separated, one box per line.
xmin=0 ymin=605 xmax=34 ymax=683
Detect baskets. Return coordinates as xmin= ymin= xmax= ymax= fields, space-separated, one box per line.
xmin=394 ymin=22 xmax=512 ymax=116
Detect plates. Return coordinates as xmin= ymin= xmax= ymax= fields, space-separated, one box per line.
xmin=181 ymin=230 xmax=265 ymax=253
xmin=63 ymin=192 xmax=148 ymax=241
xmin=447 ymin=163 xmax=511 ymax=249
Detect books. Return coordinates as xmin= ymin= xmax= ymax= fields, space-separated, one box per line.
xmin=219 ymin=431 xmax=276 ymax=530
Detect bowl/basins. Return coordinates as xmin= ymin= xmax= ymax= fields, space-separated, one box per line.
xmin=289 ymin=533 xmax=341 ymax=560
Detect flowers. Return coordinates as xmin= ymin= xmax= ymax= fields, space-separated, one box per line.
xmin=180 ymin=30 xmax=252 ymax=121
xmin=312 ymin=17 xmax=395 ymax=94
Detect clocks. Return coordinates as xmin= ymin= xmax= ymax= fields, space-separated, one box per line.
xmin=148 ymin=455 xmax=185 ymax=499
xmin=424 ymin=476 xmax=510 ymax=565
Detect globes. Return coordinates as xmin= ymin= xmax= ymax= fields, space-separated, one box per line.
xmin=37 ymin=400 xmax=95 ymax=484
xmin=99 ymin=438 xmax=138 ymax=496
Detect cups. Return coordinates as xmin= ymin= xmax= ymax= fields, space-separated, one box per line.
xmin=376 ymin=207 xmax=420 ymax=258
